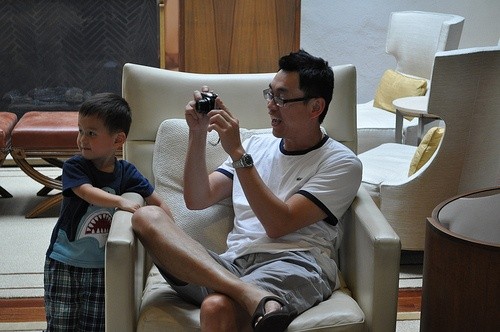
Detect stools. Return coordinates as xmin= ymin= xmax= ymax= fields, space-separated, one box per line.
xmin=11 ymin=111 xmax=79 ymax=219
xmin=0 ymin=112 xmax=18 ymax=198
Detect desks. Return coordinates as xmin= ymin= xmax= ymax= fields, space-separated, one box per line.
xmin=419 ymin=187 xmax=500 ymax=332
xmin=392 ymin=96 xmax=440 ymax=146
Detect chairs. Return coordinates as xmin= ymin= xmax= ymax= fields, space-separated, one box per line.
xmin=357 ymin=10 xmax=500 ymax=249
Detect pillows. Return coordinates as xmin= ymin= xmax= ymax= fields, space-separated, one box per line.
xmin=152 ymin=119 xmax=327 ymax=255
xmin=408 ymin=127 xmax=444 ymax=179
xmin=374 ymin=69 xmax=427 ymax=121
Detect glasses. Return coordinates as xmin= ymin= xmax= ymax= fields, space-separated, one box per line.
xmin=263 ymin=88 xmax=310 ymax=107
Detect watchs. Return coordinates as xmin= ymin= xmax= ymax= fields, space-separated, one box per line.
xmin=233 ymin=153 xmax=253 ymax=169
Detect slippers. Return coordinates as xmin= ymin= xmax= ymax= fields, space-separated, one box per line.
xmin=251 ymin=296 xmax=300 ymax=332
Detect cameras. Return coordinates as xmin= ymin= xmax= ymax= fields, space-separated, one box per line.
xmin=195 ymin=91 xmax=221 ymax=115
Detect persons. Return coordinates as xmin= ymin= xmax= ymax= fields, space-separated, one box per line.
xmin=43 ymin=93 xmax=175 ymax=332
xmin=131 ymin=50 xmax=363 ymax=332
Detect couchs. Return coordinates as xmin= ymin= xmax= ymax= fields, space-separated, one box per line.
xmin=105 ymin=63 xmax=401 ymax=332
